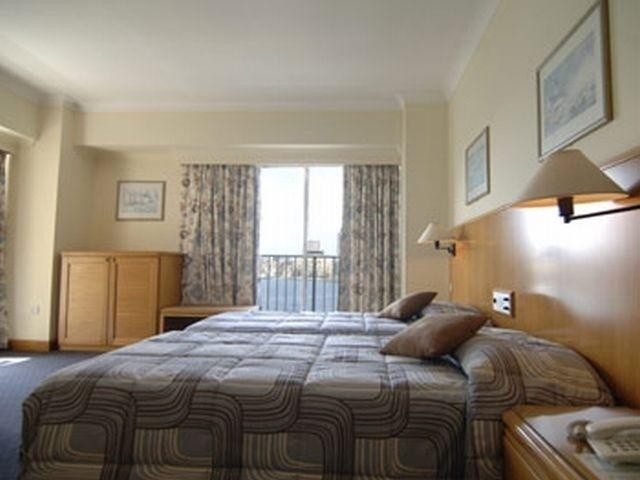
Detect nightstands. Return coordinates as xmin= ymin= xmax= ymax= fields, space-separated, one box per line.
xmin=499 ymin=403 xmax=639 ymax=480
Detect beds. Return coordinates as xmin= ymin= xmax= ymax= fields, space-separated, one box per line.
xmin=18 ymin=295 xmax=612 ymax=479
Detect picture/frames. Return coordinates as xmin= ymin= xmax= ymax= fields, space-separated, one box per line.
xmin=535 ymin=1 xmax=614 ymax=163
xmin=463 ymin=125 xmax=489 ymax=205
xmin=115 ymin=181 xmax=166 ymax=222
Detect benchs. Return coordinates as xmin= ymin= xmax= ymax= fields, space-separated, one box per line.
xmin=158 ymin=302 xmax=261 ymax=335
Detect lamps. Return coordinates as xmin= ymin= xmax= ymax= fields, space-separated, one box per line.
xmin=416 ymin=219 xmax=461 ymax=257
xmin=519 ymin=148 xmax=640 ymax=224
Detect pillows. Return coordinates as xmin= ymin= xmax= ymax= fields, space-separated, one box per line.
xmin=376 ymin=288 xmax=489 ymax=361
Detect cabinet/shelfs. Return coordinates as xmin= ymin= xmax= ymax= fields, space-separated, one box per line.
xmin=57 ymin=249 xmax=184 ymax=353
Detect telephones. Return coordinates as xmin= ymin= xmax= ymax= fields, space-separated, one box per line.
xmin=584 ymin=415 xmax=640 ymax=464
